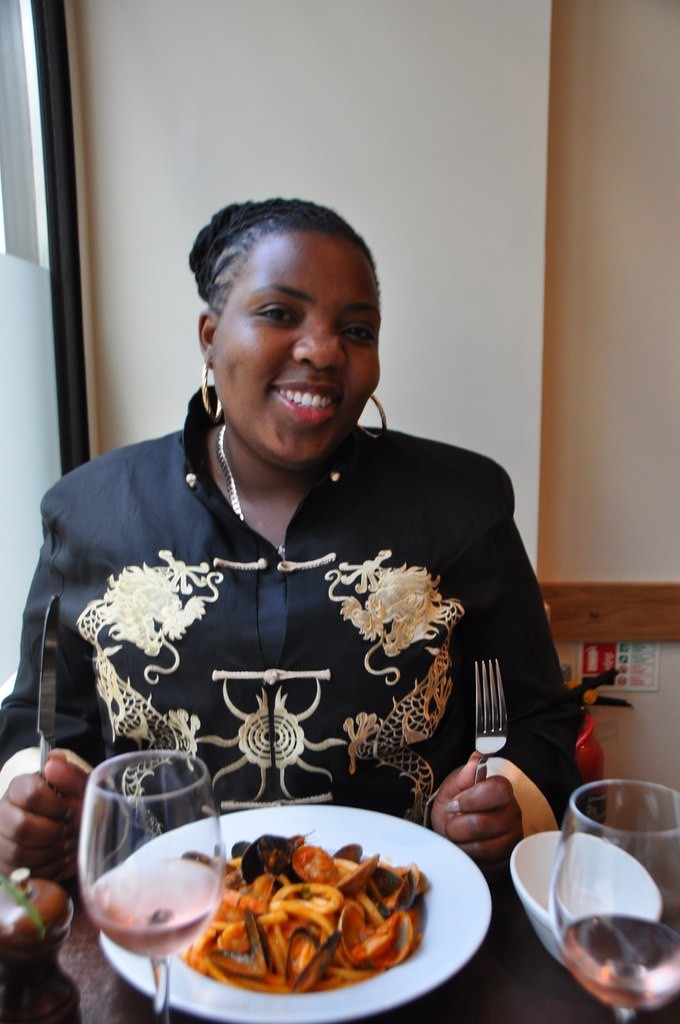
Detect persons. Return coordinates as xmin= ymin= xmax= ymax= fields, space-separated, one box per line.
xmin=3 ymin=197 xmax=588 ymax=899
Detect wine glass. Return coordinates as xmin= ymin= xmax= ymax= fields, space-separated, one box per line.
xmin=78 ymin=749 xmax=226 ymax=1024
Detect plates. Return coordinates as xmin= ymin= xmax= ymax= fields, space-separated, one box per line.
xmin=96 ymin=802 xmax=495 ymax=1023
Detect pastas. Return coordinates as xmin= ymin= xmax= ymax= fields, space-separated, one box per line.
xmin=182 ymin=834 xmax=430 ymax=992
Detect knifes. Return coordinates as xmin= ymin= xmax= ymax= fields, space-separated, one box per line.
xmin=36 ymin=593 xmax=63 ymax=781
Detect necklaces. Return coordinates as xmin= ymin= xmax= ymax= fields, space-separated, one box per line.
xmin=219 ymin=423 xmax=286 ymax=557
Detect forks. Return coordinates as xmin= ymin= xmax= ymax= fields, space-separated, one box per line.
xmin=471 ymin=658 xmax=510 ymax=786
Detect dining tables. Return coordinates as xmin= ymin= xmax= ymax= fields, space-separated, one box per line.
xmin=0 ymin=859 xmax=680 ymax=1024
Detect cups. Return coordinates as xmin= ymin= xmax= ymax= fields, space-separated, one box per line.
xmin=548 ymin=778 xmax=679 ymax=1023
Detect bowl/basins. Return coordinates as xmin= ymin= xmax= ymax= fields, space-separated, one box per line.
xmin=509 ymin=825 xmax=666 ymax=980
